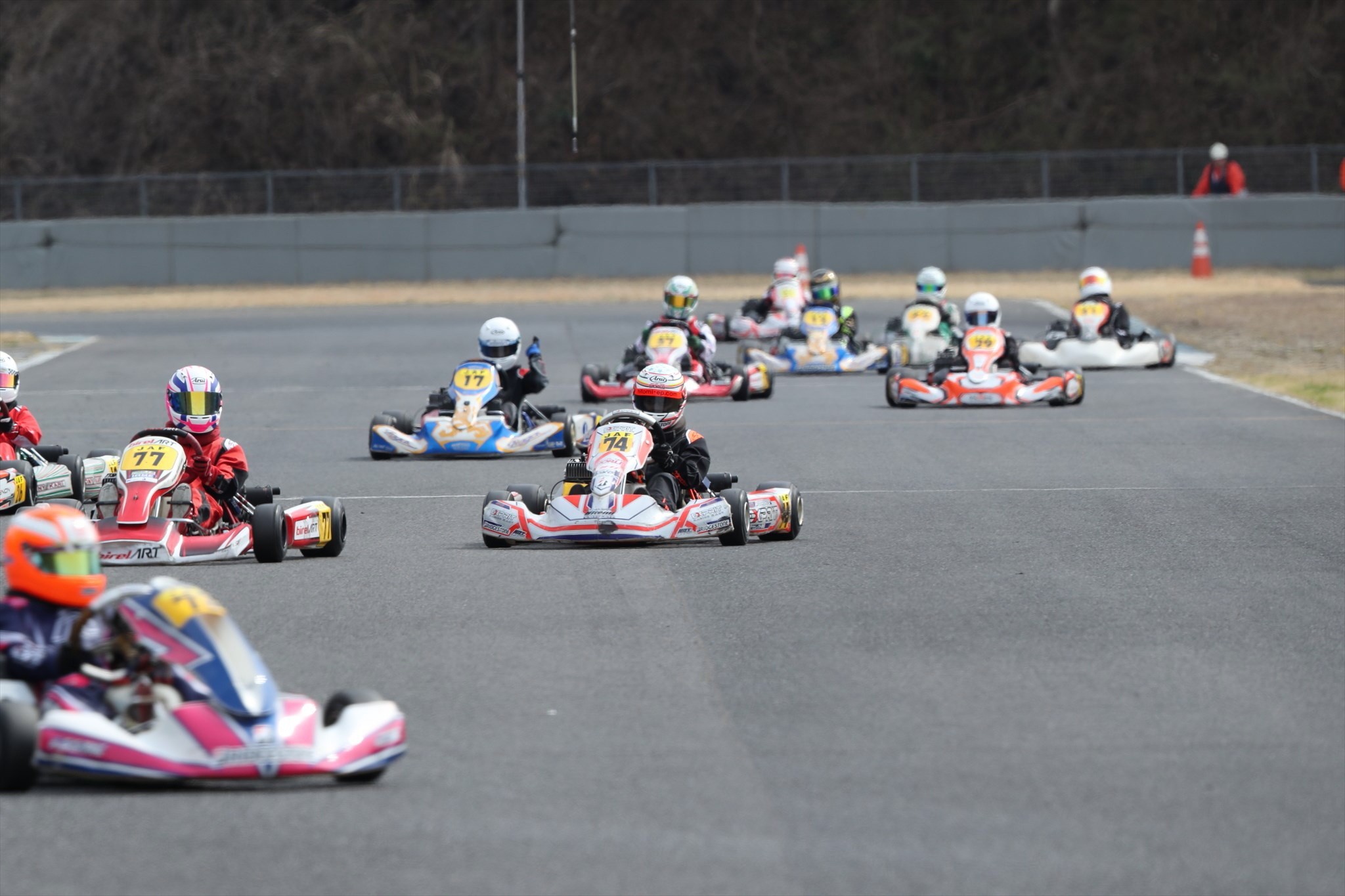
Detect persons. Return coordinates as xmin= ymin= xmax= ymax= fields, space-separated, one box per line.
xmin=897 ymin=266 xmax=963 ymax=352
xmin=437 ymin=317 xmax=550 ymax=431
xmin=1067 ymin=266 xmax=1132 ymax=346
xmin=0 ymin=501 xmax=121 ymax=694
xmin=0 ymin=351 xmax=43 ymax=462
xmin=1190 ymin=141 xmax=1246 ymax=197
xmin=615 ymin=275 xmax=718 ymax=384
xmin=787 ymin=268 xmax=858 ymax=356
xmin=757 ymin=257 xmax=812 ymax=314
xmin=569 ymin=363 xmax=711 ymax=512
xmin=934 ymin=291 xmax=1021 ymax=375
xmin=165 ymin=365 xmax=250 ymax=538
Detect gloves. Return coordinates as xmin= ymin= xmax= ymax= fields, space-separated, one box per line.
xmin=526 ymin=335 xmax=541 ymax=359
xmin=191 ymin=453 xmax=212 ymax=478
xmin=688 ymin=335 xmax=701 ymax=349
xmin=79 ymin=619 xmax=103 ymax=652
xmin=651 ymin=442 xmax=680 ymax=472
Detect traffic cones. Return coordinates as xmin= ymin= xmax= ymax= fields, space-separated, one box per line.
xmin=1190 ymin=219 xmax=1214 ymax=279
xmin=792 ymin=242 xmax=814 ymax=307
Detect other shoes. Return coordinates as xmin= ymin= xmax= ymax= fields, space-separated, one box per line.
xmin=168 ymin=482 xmax=193 ymax=534
xmin=501 ymin=401 xmax=517 ymax=427
xmin=632 ymin=486 xmax=650 ymax=497
xmin=635 ymin=356 xmax=646 ymax=371
xmin=681 ymin=353 xmax=691 ymax=370
xmin=569 ymin=484 xmax=587 ymax=495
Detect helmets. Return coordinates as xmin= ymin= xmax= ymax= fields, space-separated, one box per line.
xmin=915 ymin=266 xmax=947 ymax=301
xmin=661 ymin=276 xmax=699 ymax=319
xmin=1209 ymin=142 xmax=1228 ymax=160
xmin=772 ymin=257 xmax=799 ymax=280
xmin=630 ymin=363 xmax=688 ymax=430
xmin=1079 ymin=266 xmax=1112 ymax=299
xmin=477 ymin=317 xmax=522 ymax=371
xmin=964 ymin=291 xmax=1002 ymax=328
xmin=0 ymin=351 xmax=21 ymax=403
xmin=810 ymin=268 xmax=839 ymax=302
xmin=2 ymin=502 xmax=106 ymax=608
xmin=164 ymin=365 xmax=224 ymax=434
